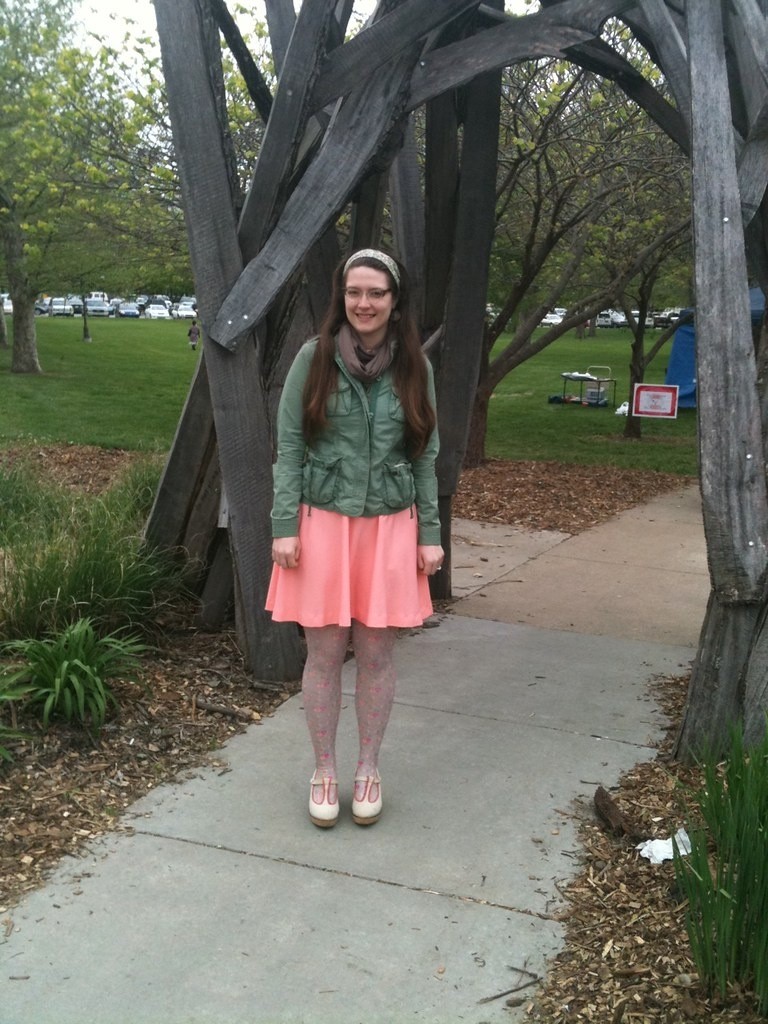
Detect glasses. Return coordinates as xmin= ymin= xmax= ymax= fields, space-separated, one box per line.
xmin=341 ymin=287 xmax=392 ymax=304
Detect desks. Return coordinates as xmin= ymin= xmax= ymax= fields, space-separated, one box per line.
xmin=562 ymin=374 xmax=617 ymax=408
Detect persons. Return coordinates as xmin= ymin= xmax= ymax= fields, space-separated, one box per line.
xmin=188 ymin=320 xmax=200 ymax=351
xmin=264 ymin=247 xmax=445 ymax=828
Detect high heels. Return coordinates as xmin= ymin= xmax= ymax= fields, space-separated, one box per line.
xmin=350 ymin=767 xmax=383 ymax=825
xmin=308 ymin=769 xmax=340 ymax=827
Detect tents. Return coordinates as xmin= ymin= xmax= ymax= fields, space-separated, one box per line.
xmin=664 ymin=287 xmax=766 ymax=410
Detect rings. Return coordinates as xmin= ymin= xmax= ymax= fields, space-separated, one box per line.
xmin=436 ymin=565 xmax=442 ymax=570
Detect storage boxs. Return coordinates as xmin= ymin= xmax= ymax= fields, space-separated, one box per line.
xmin=587 ymin=387 xmax=605 ymax=400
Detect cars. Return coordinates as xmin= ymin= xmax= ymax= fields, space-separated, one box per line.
xmin=1 ymin=289 xmax=199 ymax=319
xmin=484 ymin=302 xmax=686 ymax=328
